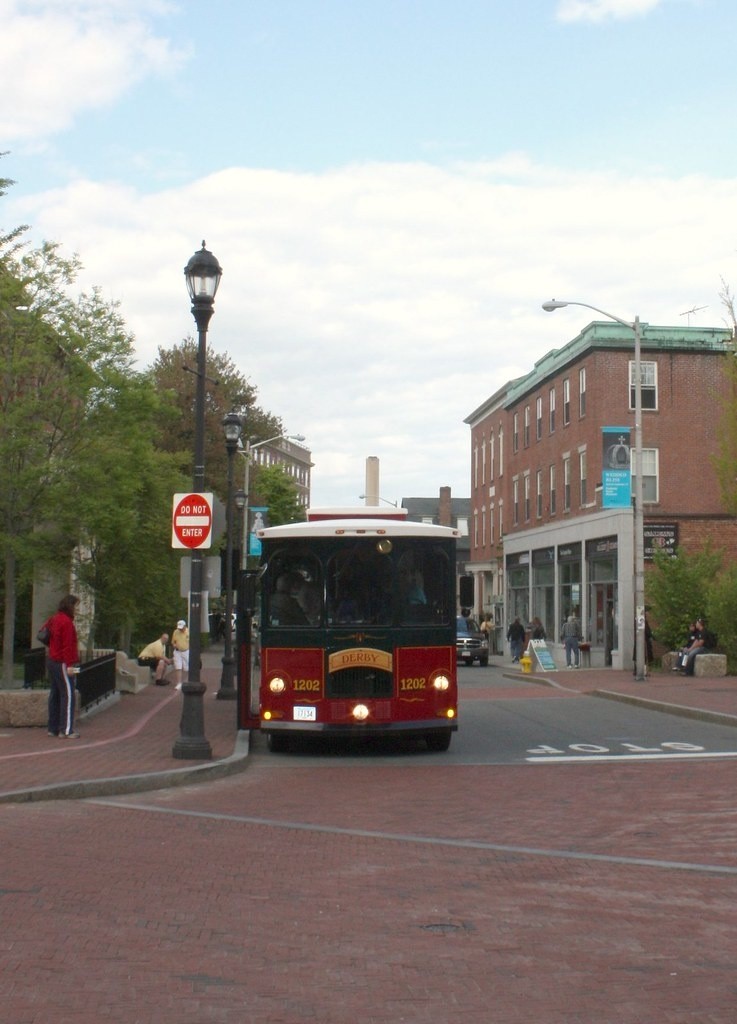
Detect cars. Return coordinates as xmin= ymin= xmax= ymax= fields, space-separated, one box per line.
xmin=455 ymin=616 xmax=489 ymax=668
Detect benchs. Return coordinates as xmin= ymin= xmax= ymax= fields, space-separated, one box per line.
xmin=662 ymin=651 xmax=728 ymax=677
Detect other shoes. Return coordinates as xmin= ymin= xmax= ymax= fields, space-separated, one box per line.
xmin=680 ymin=672 xmax=686 ymax=676
xmin=569 ymin=665 xmax=572 ymax=668
xmin=156 ymin=678 xmax=170 ymax=686
xmin=48 ymin=732 xmax=54 ymax=736
xmin=58 ymin=732 xmax=80 ymax=738
xmin=176 ymin=684 xmax=181 ymax=689
xmin=575 ymin=665 xmax=578 ymax=668
xmin=672 ymin=667 xmax=681 ymax=670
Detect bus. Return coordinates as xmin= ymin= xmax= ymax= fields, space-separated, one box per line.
xmin=242 ymin=518 xmax=475 ymax=753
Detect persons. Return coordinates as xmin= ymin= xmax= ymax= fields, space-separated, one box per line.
xmin=560 ymin=616 xmax=581 ymax=669
xmin=506 ymin=617 xmax=544 ymax=664
xmin=259 ymin=538 xmax=335 ymax=630
xmin=137 ymin=633 xmax=173 ymax=686
xmin=171 ymin=620 xmax=189 ymax=691
xmin=39 ymin=594 xmax=80 ymax=740
xmin=672 ymin=618 xmax=713 ymax=676
xmin=607 ymin=602 xmax=613 ymax=649
xmin=390 ymin=565 xmax=427 ymax=607
xmin=645 ymin=605 xmax=658 ymax=666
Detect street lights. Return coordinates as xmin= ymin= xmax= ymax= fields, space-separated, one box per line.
xmin=235 ymin=434 xmax=306 ymax=573
xmin=215 ymin=406 xmax=242 ymax=700
xmin=360 ymin=494 xmax=398 ymax=507
xmin=543 ymin=298 xmax=646 ymax=681
xmin=233 ymin=486 xmax=247 ymax=674
xmin=171 ymin=237 xmax=222 ymax=760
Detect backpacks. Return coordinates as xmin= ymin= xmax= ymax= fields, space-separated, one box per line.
xmin=711 ymin=633 xmax=717 ymax=647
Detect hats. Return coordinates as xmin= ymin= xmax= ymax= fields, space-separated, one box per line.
xmin=177 ymin=620 xmax=185 ymax=629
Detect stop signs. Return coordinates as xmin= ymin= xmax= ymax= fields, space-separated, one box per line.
xmin=174 ymin=494 xmax=212 ymax=548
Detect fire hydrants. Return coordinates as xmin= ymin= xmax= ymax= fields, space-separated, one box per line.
xmin=519 ymin=651 xmax=532 ymax=674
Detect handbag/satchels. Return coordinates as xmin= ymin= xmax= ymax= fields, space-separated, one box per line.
xmin=682 ymin=655 xmax=689 ymax=667
xmin=37 ymin=616 xmax=54 ymax=648
